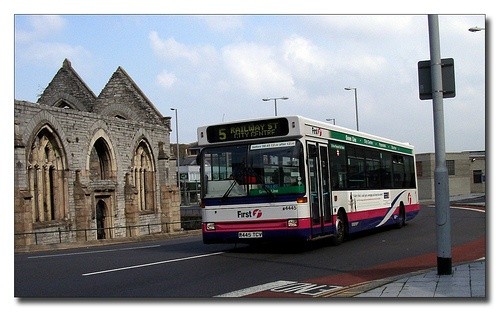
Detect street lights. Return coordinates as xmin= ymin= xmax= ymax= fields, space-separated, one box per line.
xmin=170 ymin=107 xmax=181 ymax=189
xmin=326 ymin=118 xmax=335 ymax=125
xmin=344 ymin=87 xmax=359 ymax=131
xmin=263 ymin=97 xmax=288 ymax=115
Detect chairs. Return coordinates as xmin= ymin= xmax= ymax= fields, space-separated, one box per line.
xmin=347 ymin=167 xmax=407 ymax=191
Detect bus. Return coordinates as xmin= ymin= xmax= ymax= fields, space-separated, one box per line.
xmin=197 ymin=115 xmax=420 ymax=245
